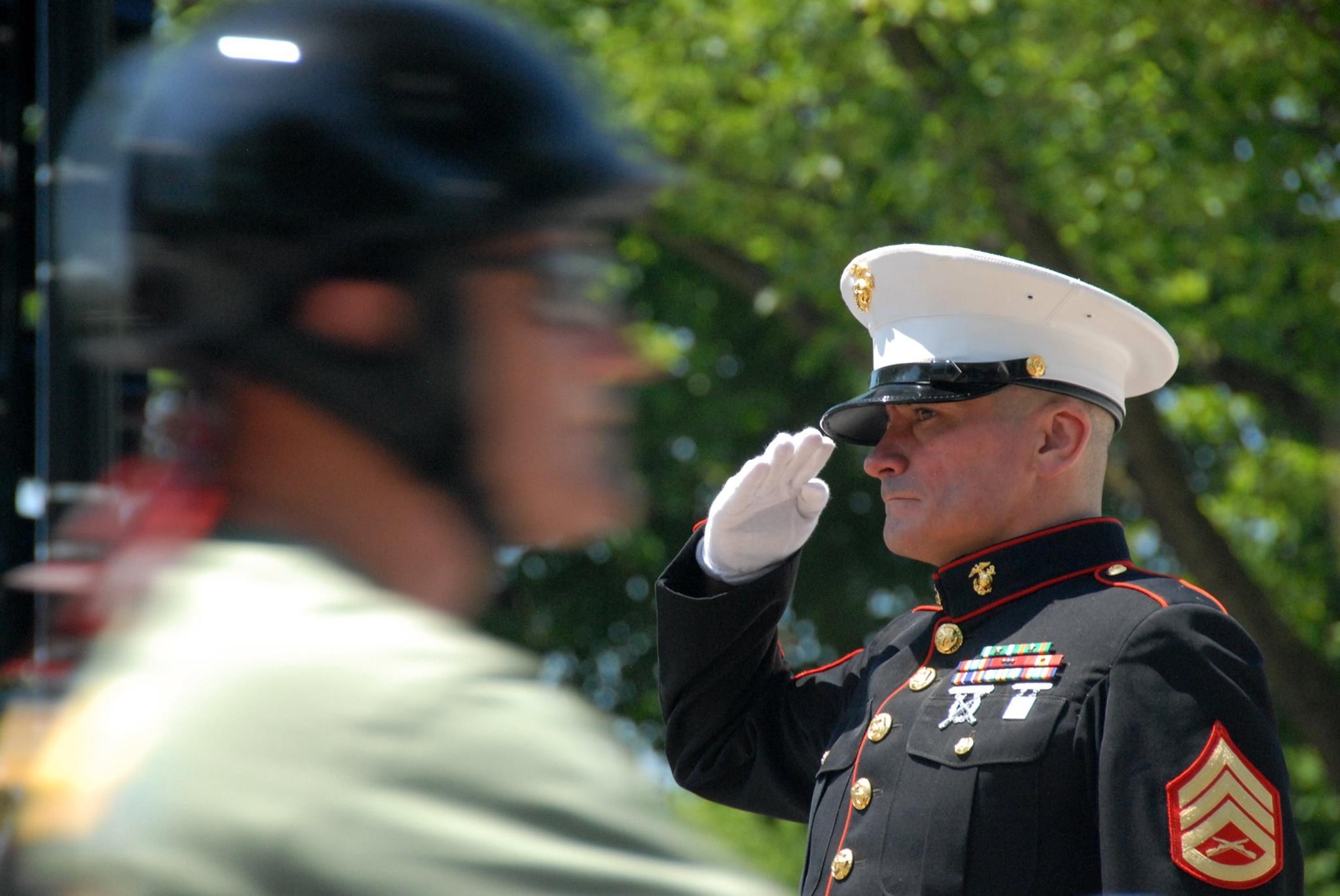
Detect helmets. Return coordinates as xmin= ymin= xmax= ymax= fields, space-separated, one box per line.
xmin=119 ymin=0 xmax=659 ymax=366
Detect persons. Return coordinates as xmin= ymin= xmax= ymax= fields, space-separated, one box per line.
xmin=655 ymin=244 xmax=1305 ymax=896
xmin=2 ymin=386 xmax=232 ymax=698
xmin=0 ymin=0 xmax=804 ymax=896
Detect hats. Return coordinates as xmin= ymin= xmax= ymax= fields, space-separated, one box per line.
xmin=817 ymin=243 xmax=1181 ymax=445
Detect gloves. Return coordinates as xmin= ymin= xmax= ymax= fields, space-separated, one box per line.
xmin=702 ymin=427 xmax=829 ymax=577
